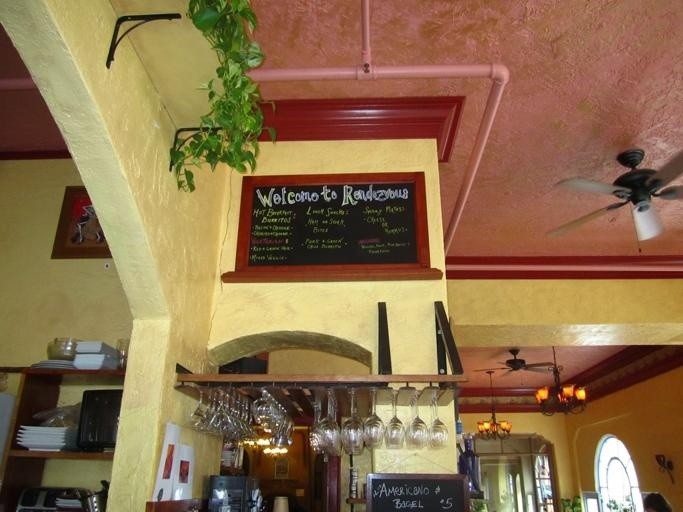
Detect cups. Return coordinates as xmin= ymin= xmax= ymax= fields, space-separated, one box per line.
xmin=272 ymin=497 xmax=290 ymax=510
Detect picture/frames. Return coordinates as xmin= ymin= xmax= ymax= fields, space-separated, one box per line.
xmin=50 ymin=185 xmax=112 ymax=259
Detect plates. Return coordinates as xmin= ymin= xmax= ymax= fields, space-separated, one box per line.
xmin=32 ymin=340 xmax=117 ymax=370
xmin=16 ymin=424 xmax=74 ymax=452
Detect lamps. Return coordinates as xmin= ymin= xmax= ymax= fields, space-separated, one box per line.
xmin=535 ymin=346 xmax=586 ymax=416
xmin=631 ymin=203 xmax=664 ymax=241
xmin=477 ymin=371 xmax=512 ymax=441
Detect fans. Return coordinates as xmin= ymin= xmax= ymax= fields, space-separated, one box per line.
xmin=473 ymin=349 xmax=555 ymax=376
xmin=547 ymin=149 xmax=683 ymax=238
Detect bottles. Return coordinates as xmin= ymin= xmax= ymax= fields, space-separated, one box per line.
xmin=459 ymin=436 xmax=481 ymax=490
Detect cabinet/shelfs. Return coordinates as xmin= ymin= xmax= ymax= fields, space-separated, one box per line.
xmin=0 ymin=367 xmax=126 ymax=512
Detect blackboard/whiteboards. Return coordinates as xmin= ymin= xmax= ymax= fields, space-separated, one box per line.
xmin=234 ymin=172 xmax=430 ymax=272
xmin=366 ymin=473 xmax=470 ymax=512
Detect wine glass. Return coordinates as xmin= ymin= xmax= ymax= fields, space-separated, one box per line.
xmin=189 ymin=383 xmax=450 ymax=456
